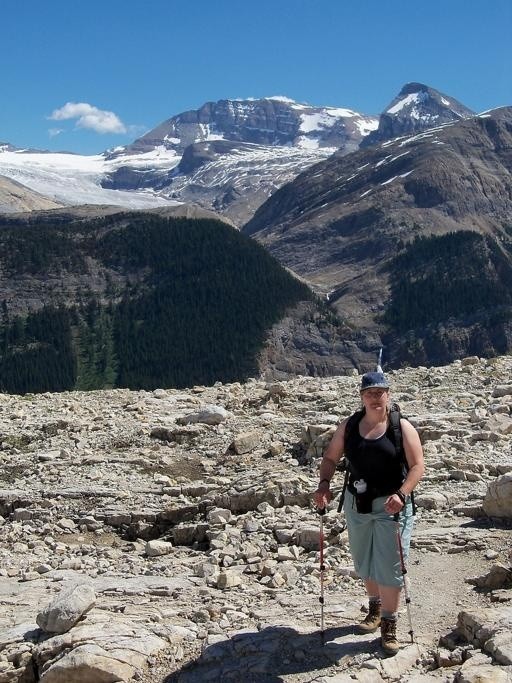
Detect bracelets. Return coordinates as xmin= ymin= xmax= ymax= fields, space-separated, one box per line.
xmin=318 ymin=478 xmax=329 ymax=488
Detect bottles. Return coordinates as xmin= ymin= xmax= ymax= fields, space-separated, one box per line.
xmin=354 ymin=479 xmax=367 ymax=494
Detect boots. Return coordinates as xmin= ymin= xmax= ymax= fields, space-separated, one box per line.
xmin=380 ymin=616 xmax=400 ymax=655
xmin=356 ymin=599 xmax=382 ymax=635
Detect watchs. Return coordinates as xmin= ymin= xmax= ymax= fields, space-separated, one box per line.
xmin=396 ymin=489 xmax=410 ymax=502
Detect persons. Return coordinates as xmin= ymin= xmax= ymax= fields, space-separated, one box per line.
xmin=312 ymin=371 xmax=424 ymax=656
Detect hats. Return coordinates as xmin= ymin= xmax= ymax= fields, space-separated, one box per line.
xmin=359 ymin=372 xmax=389 ymax=394
